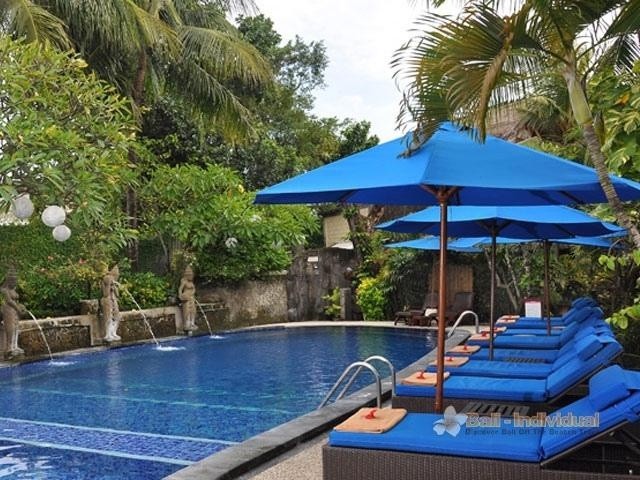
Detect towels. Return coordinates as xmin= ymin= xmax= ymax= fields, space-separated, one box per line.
xmin=500 ymin=315 xmax=520 ymax=319
xmin=428 ymin=356 xmax=469 ymax=368
xmin=448 ymin=345 xmax=480 ymax=353
xmin=468 ymin=332 xmax=497 ymax=340
xmin=424 ymin=309 xmax=437 ymax=316
xmin=482 ymin=326 xmax=507 ymax=332
xmin=401 ymin=370 xmax=450 ymax=387
xmin=497 ymin=319 xmax=515 ymax=323
xmin=334 ymin=406 xmax=407 ymax=435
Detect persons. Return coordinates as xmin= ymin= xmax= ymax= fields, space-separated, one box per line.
xmin=0 ymin=266 xmax=26 ymax=361
xmin=179 ymin=265 xmax=200 ymax=332
xmin=99 ymin=264 xmax=124 ymax=341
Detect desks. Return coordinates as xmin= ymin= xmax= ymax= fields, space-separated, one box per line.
xmin=411 ymin=314 xmax=431 ymax=325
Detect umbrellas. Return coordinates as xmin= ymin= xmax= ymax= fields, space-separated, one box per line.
xmin=447 ymin=238 xmax=626 ymax=335
xmin=381 ymin=235 xmax=485 ymax=255
xmin=373 ymin=205 xmax=632 ymax=361
xmin=251 ymin=118 xmax=640 ymax=414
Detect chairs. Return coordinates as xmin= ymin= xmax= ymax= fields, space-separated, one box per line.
xmin=391 ymin=355 xmax=623 ymax=415
xmin=394 ymin=291 xmax=438 ymax=325
xmin=423 ymin=341 xmax=615 ymax=379
xmin=467 ymin=296 xmax=603 ymax=349
xmin=321 ymin=365 xmax=640 ymax=480
xmin=446 ymin=332 xmax=609 ymax=363
xmin=427 ymin=291 xmax=475 ymax=327
